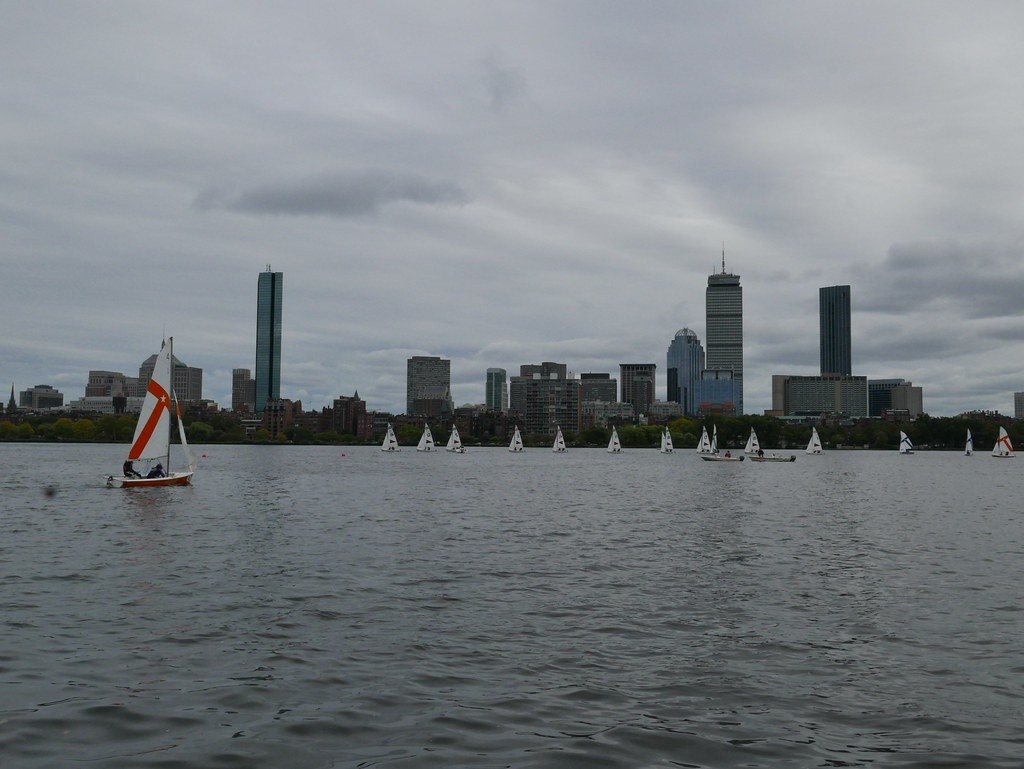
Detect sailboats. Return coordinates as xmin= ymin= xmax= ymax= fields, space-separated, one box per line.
xmin=607 ymin=425 xmax=625 ymax=454
xmin=710 ymin=424 xmax=720 ymax=455
xmin=417 ymin=423 xmax=438 ymax=452
xmin=102 ymin=336 xmax=195 ymax=488
xmin=381 ymin=422 xmax=401 ymax=452
xmin=552 ymin=425 xmax=569 ymax=453
xmin=665 ymin=426 xmax=675 ymax=454
xmin=661 ymin=431 xmax=667 ymax=453
xmin=508 ymin=425 xmax=527 ymax=453
xmin=447 ymin=424 xmax=467 ymax=453
xmin=697 ymin=426 xmax=710 ymax=453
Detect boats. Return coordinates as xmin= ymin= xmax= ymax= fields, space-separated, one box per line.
xmin=749 ymin=455 xmax=796 ymax=462
xmin=806 ymin=427 xmax=825 ymax=455
xmin=965 ymin=428 xmax=974 ymax=456
xmin=899 ymin=431 xmax=915 ymax=454
xmin=701 ymin=456 xmax=745 ymax=461
xmin=992 ymin=426 xmax=1016 ymax=458
xmin=745 ymin=427 xmax=760 ymax=455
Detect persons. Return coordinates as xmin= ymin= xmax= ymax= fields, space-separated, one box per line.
xmin=758 ymin=449 xmax=764 ymax=457
xmin=146 ymin=464 xmax=165 ymax=479
xmin=725 ymin=450 xmax=731 ymax=458
xmin=123 ymin=459 xmax=141 ymax=479
